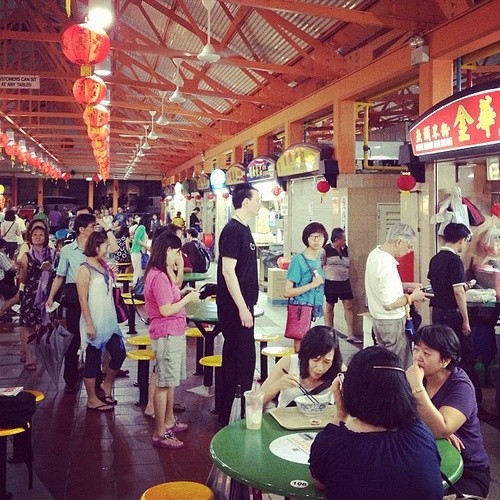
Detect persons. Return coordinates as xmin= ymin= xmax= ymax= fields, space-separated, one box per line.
xmin=308 ymin=346 xmax=444 ymax=500
xmin=251 ymin=325 xmax=349 ymax=500
xmin=365 ymin=224 xmax=434 ymax=371
xmin=0 ymin=202 xmax=206 ymax=377
xmin=404 ymin=324 xmax=491 ymax=500
xmin=427 ymin=223 xmax=482 ymax=403
xmin=45 ymin=214 xmax=130 ymax=393
xmin=145 ymin=226 xmax=201 ymax=448
xmin=216 ymin=184 xmax=261 ymax=427
xmin=323 ymin=227 xmax=363 ymax=343
xmin=283 ymin=222 xmax=327 ymax=354
xmin=77 ymin=232 xmax=126 ymax=411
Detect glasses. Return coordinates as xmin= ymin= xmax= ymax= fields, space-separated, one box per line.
xmin=310 ymin=234 xmax=325 ymax=240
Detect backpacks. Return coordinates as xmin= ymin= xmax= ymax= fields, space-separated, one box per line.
xmin=191 ymin=240 xmax=210 ymax=273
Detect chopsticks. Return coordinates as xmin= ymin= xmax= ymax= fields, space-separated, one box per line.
xmin=282 ymin=368 xmax=320 ymax=404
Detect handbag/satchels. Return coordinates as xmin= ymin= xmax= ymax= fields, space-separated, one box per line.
xmin=462 ymin=196 xmax=485 ymax=226
xmin=125 ymin=237 xmax=130 ymax=251
xmin=99 ymin=259 xmax=129 ymax=323
xmin=284 ymin=303 xmax=313 ymax=341
xmin=0 ymin=277 xmax=19 ymax=298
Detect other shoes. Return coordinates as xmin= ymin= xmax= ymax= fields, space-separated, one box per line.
xmin=117 ymin=370 xmax=129 ymax=377
xmin=26 ymin=363 xmax=37 ymax=371
xmin=6 ymin=309 xmax=18 ymax=315
xmin=64 ymin=385 xmax=73 ymax=392
xmin=0 ymin=317 xmax=12 ymax=323
xmin=347 ymin=339 xmax=362 ymax=343
xmin=174 ymin=403 xmax=185 ymax=412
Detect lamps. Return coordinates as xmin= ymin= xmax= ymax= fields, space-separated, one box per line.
xmin=0 ymin=127 xmax=59 ymax=180
xmin=408 ymin=35 xmax=430 ymax=67
xmin=85 ymin=0 xmax=221 ymax=180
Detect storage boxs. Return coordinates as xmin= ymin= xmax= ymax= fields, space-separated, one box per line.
xmin=475 ymin=268 xmax=500 ymax=300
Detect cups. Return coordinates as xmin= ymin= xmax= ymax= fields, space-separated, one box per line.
xmin=244 ymin=390 xmax=266 ymax=430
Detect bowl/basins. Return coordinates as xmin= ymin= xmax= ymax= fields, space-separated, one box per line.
xmin=294 ymin=395 xmax=331 ymax=417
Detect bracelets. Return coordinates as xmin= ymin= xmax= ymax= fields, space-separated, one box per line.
xmin=410 ymin=385 xmax=426 ymax=395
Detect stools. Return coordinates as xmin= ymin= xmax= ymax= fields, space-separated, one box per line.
xmin=109 ymin=262 xmax=296 ymax=410
xmin=141 ymin=481 xmax=215 ymax=500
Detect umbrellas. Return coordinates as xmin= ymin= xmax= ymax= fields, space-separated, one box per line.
xmin=403 ymin=294 xmax=416 ymax=338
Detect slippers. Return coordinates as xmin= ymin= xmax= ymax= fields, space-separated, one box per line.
xmin=86 ymin=404 xmax=115 ymax=411
xmin=167 ymin=415 xmax=188 ymax=432
xmin=98 ymin=394 xmax=117 ymax=404
xmin=153 ymin=430 xmax=184 ymax=449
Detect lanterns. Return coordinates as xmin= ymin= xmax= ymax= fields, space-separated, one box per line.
xmin=222 ymin=192 xmax=229 ymax=199
xmin=272 ymin=186 xmax=281 ymax=196
xmin=196 ymin=195 xmax=201 ymax=202
xmin=317 ymin=181 xmax=330 ymax=203
xmin=187 ymin=194 xmax=191 ymax=200
xmin=397 ymin=172 xmax=416 ymax=197
xmin=208 ymin=193 xmax=213 ymax=202
xmin=0 ymin=21 xmax=110 ymax=186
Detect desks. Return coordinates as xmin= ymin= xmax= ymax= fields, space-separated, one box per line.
xmin=182 ymin=273 xmax=212 ymax=282
xmin=24 ymin=389 xmax=45 ymax=402
xmin=267 ymin=268 xmax=289 ymax=307
xmin=0 ymin=421 xmax=30 ymax=500
xmin=185 ymin=302 xmax=264 ymax=387
xmin=209 ymin=413 xmax=463 ymax=500
xmin=425 ymin=293 xmax=500 ymax=366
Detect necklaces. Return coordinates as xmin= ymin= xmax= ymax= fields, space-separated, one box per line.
xmin=235 ymin=213 xmax=244 ymax=224
